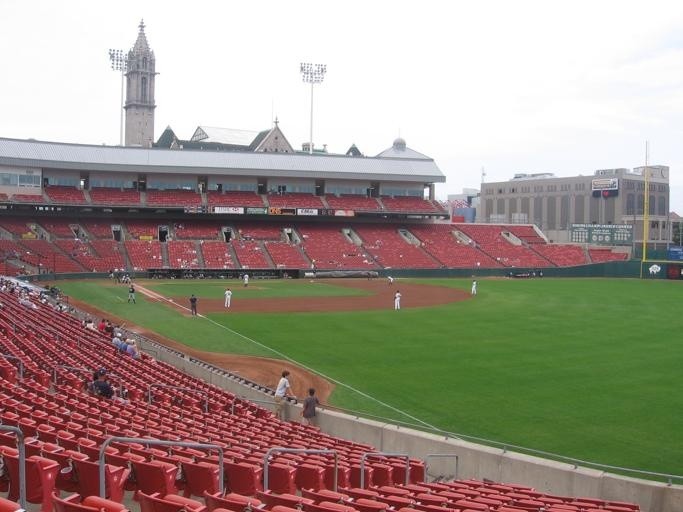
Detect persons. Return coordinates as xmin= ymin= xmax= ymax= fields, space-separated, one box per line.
xmin=224 ymin=287 xmax=232 ymax=308
xmin=126 ymin=339 xmax=141 ymax=360
xmin=188 ymin=295 xmax=197 ymax=315
xmin=94 ymin=367 xmax=114 ymax=398
xmin=0 ymin=270 xmax=132 ymax=352
xmin=470 ymin=280 xmax=477 ymax=295
xmin=393 ymin=290 xmax=401 ymax=310
xmin=242 ymin=273 xmax=249 ymax=286
xmin=272 ymin=370 xmax=297 ymax=418
xmin=150 ymin=270 xmax=294 ymax=280
xmin=87 ymin=370 xmax=99 ymax=392
xmin=298 ymin=387 xmax=319 ymax=426
xmin=386 ymin=276 xmax=393 ymax=288
xmin=126 ymin=284 xmax=136 ymax=304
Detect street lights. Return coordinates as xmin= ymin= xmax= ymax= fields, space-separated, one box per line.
xmin=300 ymin=63 xmax=327 ymax=154
xmin=109 ymin=49 xmax=136 ymax=146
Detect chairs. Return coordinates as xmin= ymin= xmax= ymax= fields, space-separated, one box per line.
xmin=2 ymin=185 xmax=452 ymax=217
xmin=0 ymin=218 xmax=629 ymax=281
xmin=2 ymin=393 xmax=640 ymax=511
xmin=0 ymin=282 xmax=235 ymax=392
